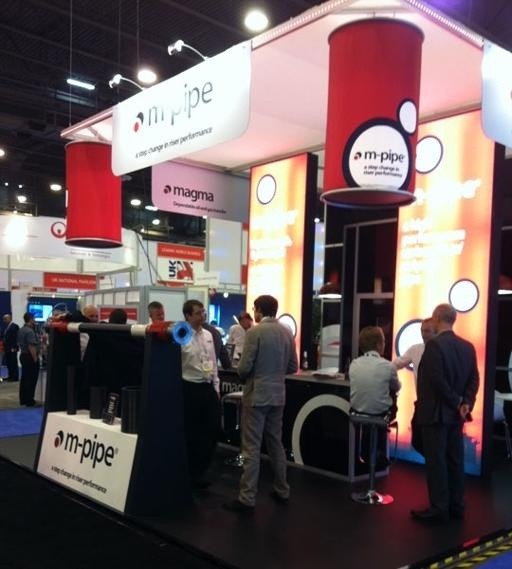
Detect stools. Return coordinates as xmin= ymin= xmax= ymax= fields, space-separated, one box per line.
xmin=221 ymin=391 xmax=248 ymax=467
xmin=348 ymin=407 xmax=399 ymax=506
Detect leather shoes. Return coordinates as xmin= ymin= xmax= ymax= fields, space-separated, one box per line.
xmin=219 ymin=501 xmax=256 ymax=514
xmin=269 ymin=490 xmax=289 ymax=507
xmin=411 ymin=508 xmax=452 ymax=527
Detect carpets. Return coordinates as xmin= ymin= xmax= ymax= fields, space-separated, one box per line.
xmin=0 ymin=364 xmax=23 ymax=382
xmin=0 ymin=406 xmax=45 ymax=437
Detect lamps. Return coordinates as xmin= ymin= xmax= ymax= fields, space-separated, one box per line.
xmin=111 ymin=72 xmax=146 ymax=93
xmin=166 ymin=37 xmax=208 ymax=61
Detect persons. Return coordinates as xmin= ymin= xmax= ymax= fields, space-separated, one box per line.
xmin=411 ymin=304 xmax=480 ymax=523
xmin=38 ymin=302 xmax=257 ymax=369
xmin=181 ymin=298 xmax=221 ymax=485
xmin=348 ymin=326 xmax=400 ymax=468
xmin=224 ymin=295 xmax=297 ymax=513
xmin=2 ymin=313 xmax=19 ymax=381
xmin=391 ymin=319 xmax=437 ymax=456
xmin=17 ymin=312 xmax=40 ymax=405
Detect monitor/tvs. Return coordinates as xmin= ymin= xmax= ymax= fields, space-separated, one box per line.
xmin=27 ymin=302 xmax=54 ymax=324
xmin=208 ymin=303 xmax=221 ymax=327
